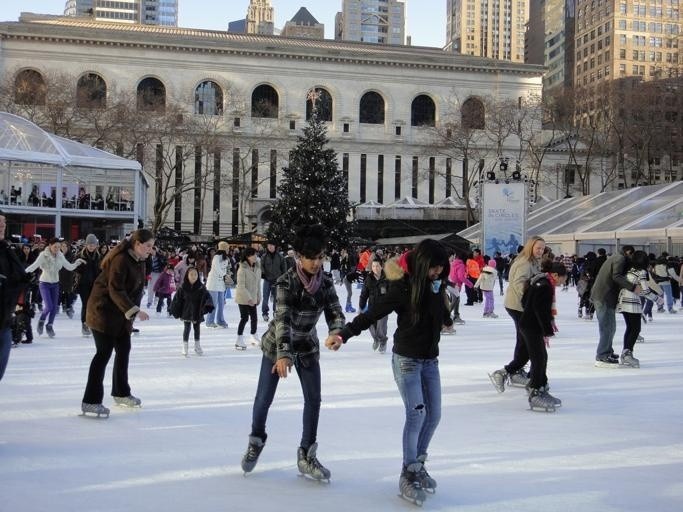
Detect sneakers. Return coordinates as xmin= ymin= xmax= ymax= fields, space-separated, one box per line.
xmin=206 ymin=323 xmax=218 ymax=328
xmin=194 ymin=341 xmax=203 ymax=354
xmin=235 ymin=333 xmax=247 ymax=348
xmin=182 ymin=341 xmax=189 ymax=355
xmin=80 ymin=398 xmax=111 ymax=416
xmin=642 ymin=307 xmax=677 ymax=323
xmin=577 ymin=307 xmax=594 ymax=319
xmin=596 ymin=351 xmax=640 ymax=366
xmin=20 ymin=337 xmax=32 ymax=343
xmin=493 ymin=365 xmax=562 ymax=408
xmin=441 ymin=298 xmax=498 ymax=333
xmin=37 ymin=320 xmax=56 ymax=336
xmin=345 ymin=301 xmax=357 ymax=312
xmin=636 ymin=335 xmax=644 ymax=341
xmin=372 ymin=337 xmax=388 ymax=352
xmin=80 ymin=323 xmax=91 ymax=334
xmin=250 ymin=334 xmax=261 ymax=345
xmin=112 ymin=392 xmax=141 ymax=406
xmin=218 ymin=321 xmax=227 ymax=327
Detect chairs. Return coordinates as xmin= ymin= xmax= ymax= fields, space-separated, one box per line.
xmin=0 ymin=190 xmax=133 ymax=211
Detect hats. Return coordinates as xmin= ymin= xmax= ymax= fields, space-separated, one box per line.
xmin=217 ymin=241 xmax=229 ymax=251
xmin=85 ymin=234 xmax=99 ymax=244
xmin=488 ymin=260 xmax=497 ymax=267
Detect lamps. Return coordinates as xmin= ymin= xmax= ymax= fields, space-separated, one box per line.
xmin=486 ymin=161 xmax=520 ymax=183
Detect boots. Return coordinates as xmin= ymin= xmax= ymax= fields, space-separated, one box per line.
xmin=416 ymin=454 xmax=436 ymax=490
xmin=295 ymin=442 xmax=331 ymax=478
xmin=240 ymin=431 xmax=268 ymax=473
xmin=398 ymin=462 xmax=426 ymax=503
